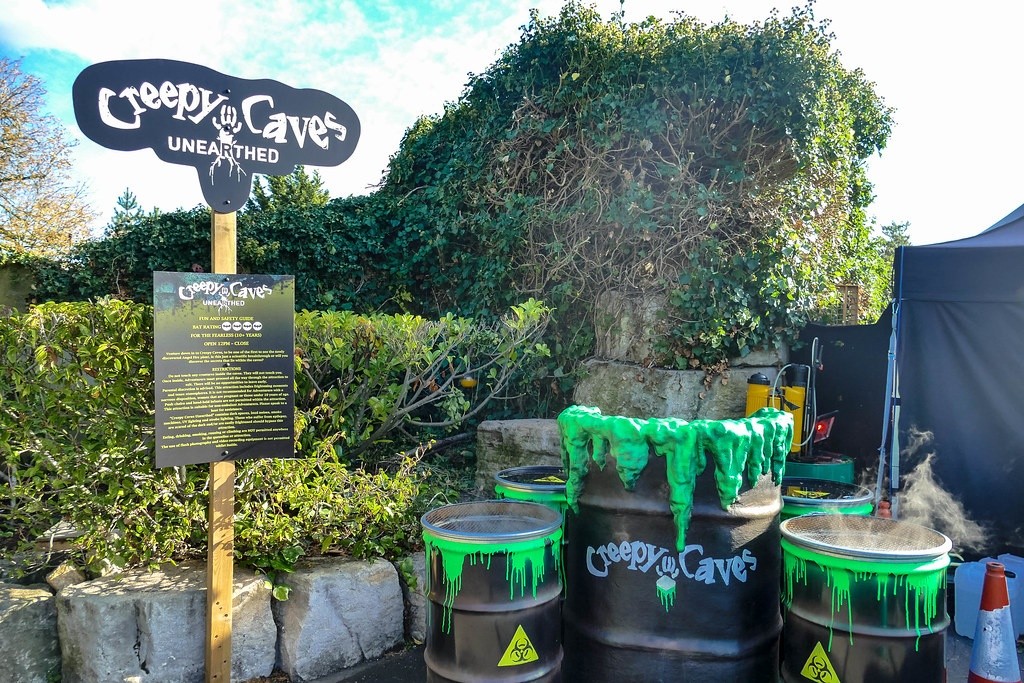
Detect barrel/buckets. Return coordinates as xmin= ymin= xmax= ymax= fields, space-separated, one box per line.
xmin=413 ymin=409 xmax=959 ymax=683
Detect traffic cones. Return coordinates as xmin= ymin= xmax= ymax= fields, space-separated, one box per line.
xmin=969 ymin=560 xmax=1023 ymax=682
